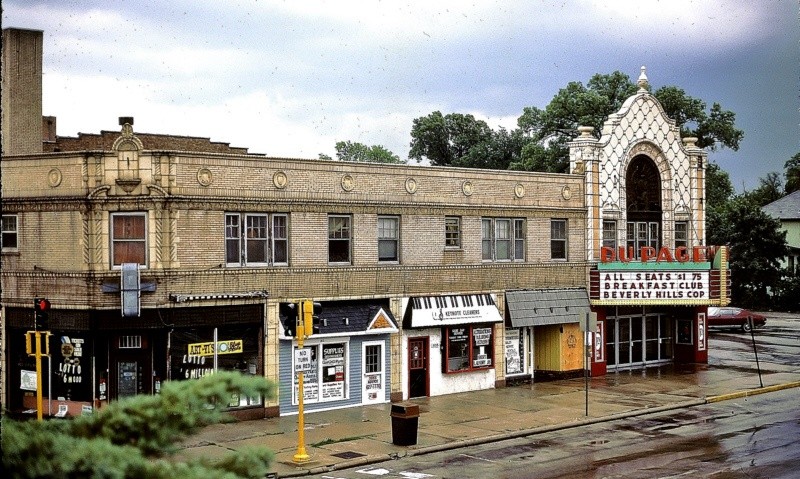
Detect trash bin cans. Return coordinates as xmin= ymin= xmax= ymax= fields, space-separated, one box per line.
xmin=390 ymin=402 xmax=421 ymax=446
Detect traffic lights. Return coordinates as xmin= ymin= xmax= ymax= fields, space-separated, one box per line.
xmin=47 ymin=333 xmax=56 ymax=355
xmin=303 ymin=301 xmax=321 ymax=335
xmin=280 ymin=302 xmax=298 ymax=337
xmin=34 ymin=297 xmax=49 ymax=331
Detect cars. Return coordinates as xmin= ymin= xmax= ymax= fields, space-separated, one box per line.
xmin=707 ymin=306 xmax=767 ymax=332
